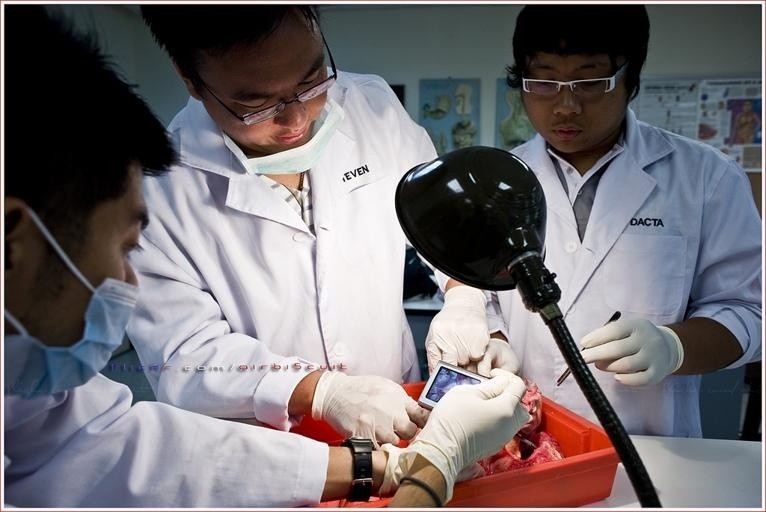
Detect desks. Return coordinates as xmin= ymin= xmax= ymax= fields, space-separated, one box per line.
xmin=580 ymin=434 xmax=762 ymax=509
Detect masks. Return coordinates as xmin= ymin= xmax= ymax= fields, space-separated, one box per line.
xmin=6 ymin=212 xmax=140 ymax=395
xmin=220 ymin=97 xmax=348 ymax=174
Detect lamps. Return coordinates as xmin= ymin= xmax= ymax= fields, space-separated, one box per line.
xmin=395 ymin=146 xmax=662 ymax=508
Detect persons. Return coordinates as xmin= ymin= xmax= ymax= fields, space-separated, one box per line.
xmin=4 ymin=4 xmax=762 ymax=508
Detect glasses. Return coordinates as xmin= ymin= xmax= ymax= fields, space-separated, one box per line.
xmin=521 ymin=65 xmax=627 ymax=100
xmin=202 ymin=29 xmax=338 ymax=126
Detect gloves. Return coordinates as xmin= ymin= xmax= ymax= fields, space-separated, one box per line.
xmin=425 ymin=286 xmax=519 ymax=378
xmin=311 ymin=368 xmax=532 ymax=504
xmin=580 ymin=318 xmax=684 ymax=387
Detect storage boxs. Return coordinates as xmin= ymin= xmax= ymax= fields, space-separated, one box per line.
xmin=256 ymin=382 xmax=622 ymax=508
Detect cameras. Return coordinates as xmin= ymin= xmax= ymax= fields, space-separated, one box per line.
xmin=417 ymin=359 xmax=491 ymax=410
